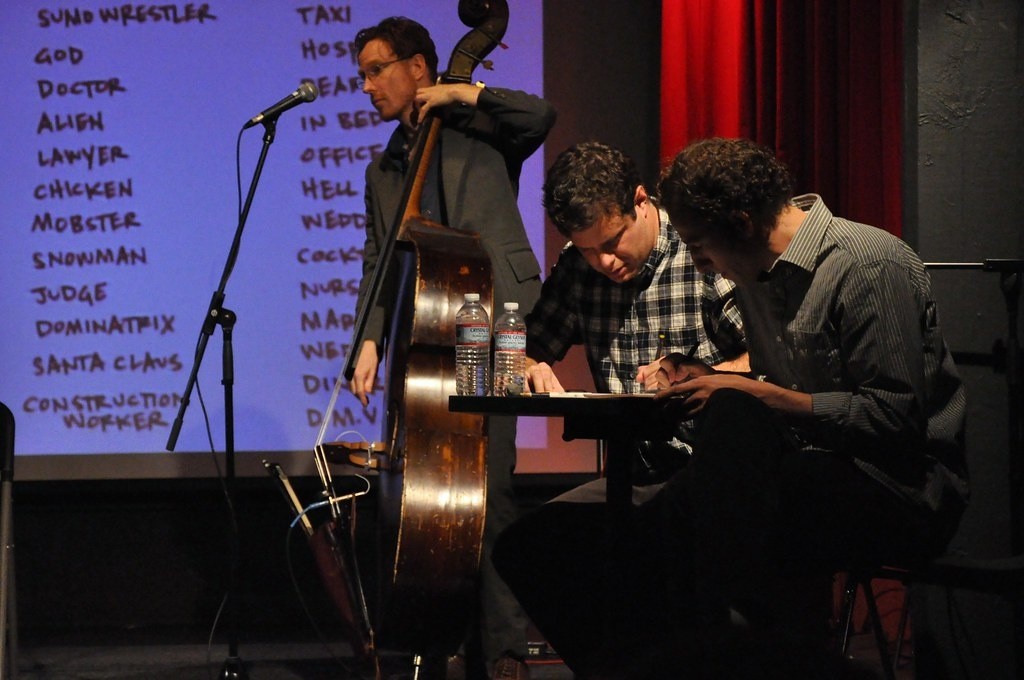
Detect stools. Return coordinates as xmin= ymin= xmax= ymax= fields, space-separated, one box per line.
xmin=838 ymin=564 xmax=957 ymax=680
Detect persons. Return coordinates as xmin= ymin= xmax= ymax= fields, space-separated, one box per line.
xmin=347 ymin=15 xmax=557 ymax=680
xmin=490 ymin=139 xmax=969 ymax=680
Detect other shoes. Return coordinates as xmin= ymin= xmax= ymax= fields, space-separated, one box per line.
xmin=492 ymin=656 xmax=530 ymax=680
xmin=447 ymin=655 xmax=466 ymax=680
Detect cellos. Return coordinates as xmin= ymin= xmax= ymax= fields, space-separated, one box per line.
xmin=312 ymin=0 xmax=510 ymax=660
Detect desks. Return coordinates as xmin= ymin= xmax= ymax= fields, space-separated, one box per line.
xmin=446 ymin=392 xmax=692 ymax=680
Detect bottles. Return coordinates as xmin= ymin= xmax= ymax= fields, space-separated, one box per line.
xmin=455 ymin=293 xmax=493 ymax=396
xmin=492 ymin=303 xmax=527 ymax=397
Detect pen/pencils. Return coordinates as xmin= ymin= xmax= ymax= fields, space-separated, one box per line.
xmin=671 ymin=341 xmax=701 ymax=387
xmin=520 ymin=392 xmax=592 ymax=397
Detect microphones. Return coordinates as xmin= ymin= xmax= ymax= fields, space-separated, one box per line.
xmin=243 ymin=82 xmax=319 ymax=129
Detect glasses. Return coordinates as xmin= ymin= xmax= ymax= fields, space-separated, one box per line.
xmin=355 ymin=55 xmax=413 ymax=89
